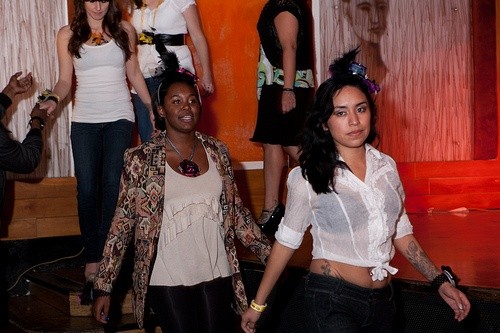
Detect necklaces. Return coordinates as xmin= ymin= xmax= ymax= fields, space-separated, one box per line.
xmin=86 ymin=22 xmax=107 ymax=45
xmin=164 ymin=132 xmax=201 ymax=178
xmin=139 ymin=1 xmax=160 ymax=43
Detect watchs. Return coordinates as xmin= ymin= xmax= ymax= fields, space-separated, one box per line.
xmin=26 ymin=117 xmax=45 ymax=131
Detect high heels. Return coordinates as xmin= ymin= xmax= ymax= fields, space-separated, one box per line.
xmin=253 ymin=203 xmax=286 ymax=231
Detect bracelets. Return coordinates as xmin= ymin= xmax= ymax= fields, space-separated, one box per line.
xmin=37 ymin=89 xmax=60 ymax=106
xmin=430 ymin=266 xmax=459 ymax=291
xmin=283 ymin=88 xmax=294 ymax=91
xmin=250 ymin=298 xmax=267 ymax=313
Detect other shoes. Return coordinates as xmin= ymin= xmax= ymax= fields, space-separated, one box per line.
xmin=85 ymin=263 xmax=99 ymax=284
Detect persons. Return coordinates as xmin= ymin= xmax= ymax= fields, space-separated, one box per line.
xmin=128 ymin=0 xmax=214 ymax=145
xmin=90 ymin=69 xmax=289 ymax=333
xmin=249 ymin=0 xmax=315 ymax=229
xmin=39 ymin=0 xmax=161 ymax=305
xmin=0 ymin=71 xmax=46 ymax=333
xmin=239 ymin=73 xmax=471 ymax=333
